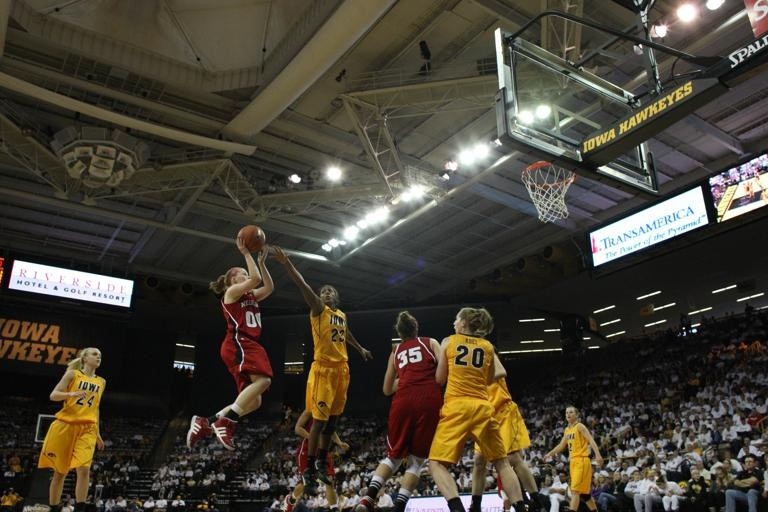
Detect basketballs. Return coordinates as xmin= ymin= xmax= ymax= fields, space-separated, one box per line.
xmin=238 ymin=224 xmax=266 ymax=253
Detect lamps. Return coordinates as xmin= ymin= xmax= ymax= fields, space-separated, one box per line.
xmin=264 ymin=165 xmax=344 ymax=189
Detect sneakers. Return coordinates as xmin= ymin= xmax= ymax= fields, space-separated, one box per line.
xmin=211 ymin=414 xmax=237 ymax=451
xmin=301 ymin=466 xmax=319 ymax=487
xmin=187 ymin=415 xmax=214 ymax=449
xmin=315 ymin=458 xmax=332 ymax=485
xmin=283 ymin=494 xmax=296 ymax=512
xmin=352 ymin=498 xmax=374 ymax=512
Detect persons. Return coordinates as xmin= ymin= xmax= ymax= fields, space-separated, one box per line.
xmin=267 ymin=243 xmax=375 ymax=485
xmin=185 ymin=237 xmax=275 ymax=450
